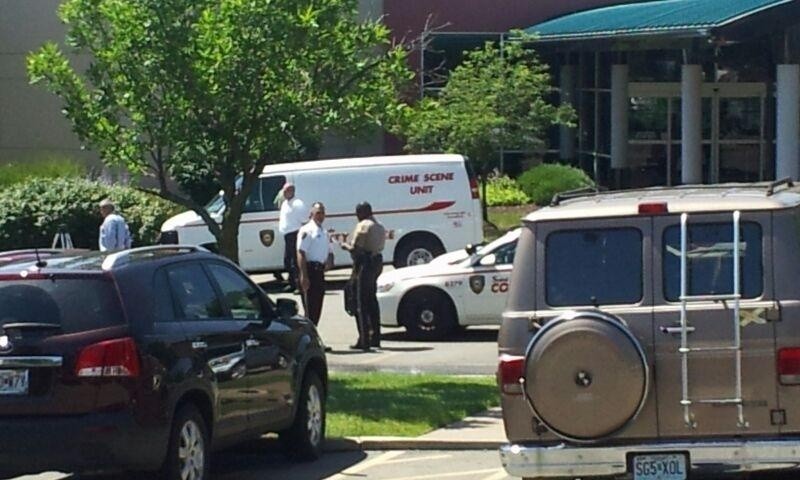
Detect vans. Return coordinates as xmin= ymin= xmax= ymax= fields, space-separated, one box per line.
xmin=161 ymin=154 xmax=485 ymax=271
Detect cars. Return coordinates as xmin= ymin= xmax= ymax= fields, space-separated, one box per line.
xmin=375 ymin=229 xmax=523 ymax=341
xmin=0 ymin=242 xmax=328 ymax=479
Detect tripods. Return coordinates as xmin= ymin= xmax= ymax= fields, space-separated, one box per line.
xmin=52 ymin=233 xmax=75 ymax=250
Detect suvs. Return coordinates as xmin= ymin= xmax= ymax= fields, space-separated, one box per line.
xmin=497 ymin=179 xmax=800 ymax=480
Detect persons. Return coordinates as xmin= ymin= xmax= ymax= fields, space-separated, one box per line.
xmin=277 ymin=183 xmax=303 ymax=295
xmin=97 ymin=199 xmax=132 ymax=252
xmin=295 ymin=198 xmax=336 ymax=354
xmin=339 ymin=200 xmax=388 ymax=351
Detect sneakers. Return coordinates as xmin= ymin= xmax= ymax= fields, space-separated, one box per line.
xmin=281 ymin=284 xmax=300 ymax=294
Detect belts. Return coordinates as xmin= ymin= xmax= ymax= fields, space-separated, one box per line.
xmin=307 ymin=262 xmax=323 ymax=270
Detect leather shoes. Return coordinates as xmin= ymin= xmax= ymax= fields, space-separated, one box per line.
xmin=348 ymin=335 xmax=380 ymax=349
